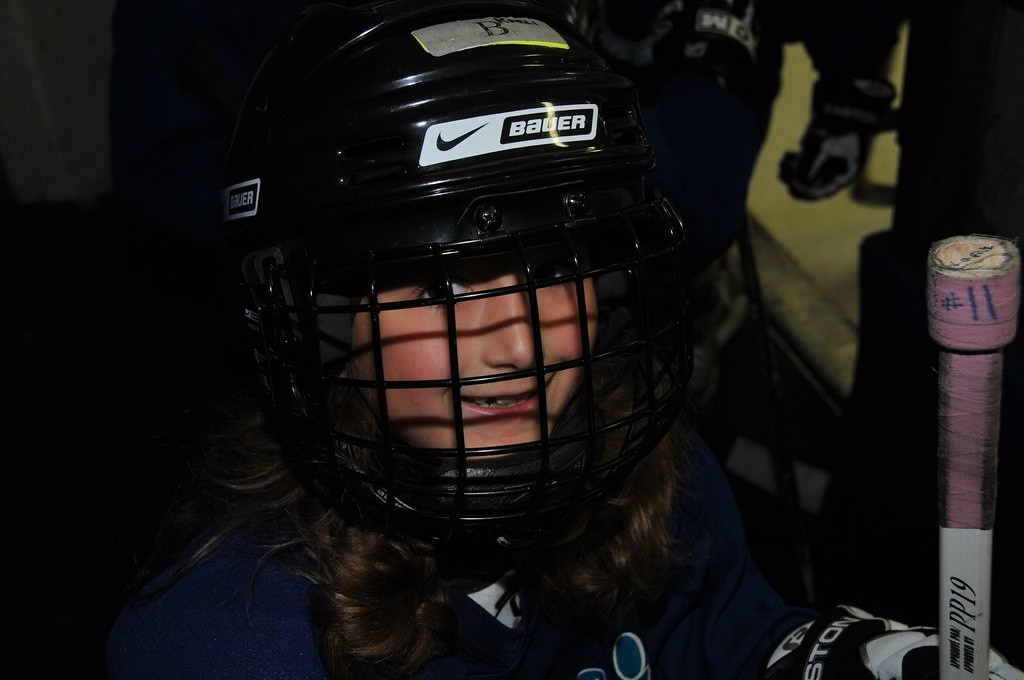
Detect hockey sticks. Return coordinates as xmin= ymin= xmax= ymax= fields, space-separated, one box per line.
xmin=920 ymin=234 xmax=1021 ymax=679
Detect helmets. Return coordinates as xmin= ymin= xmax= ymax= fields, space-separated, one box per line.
xmin=219 ymin=0 xmax=699 ymax=553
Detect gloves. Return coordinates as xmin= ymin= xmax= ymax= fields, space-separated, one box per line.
xmin=758 ymin=603 xmax=1024 ymax=680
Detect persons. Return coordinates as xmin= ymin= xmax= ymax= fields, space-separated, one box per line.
xmin=106 ymin=2 xmax=1024 ymax=680
